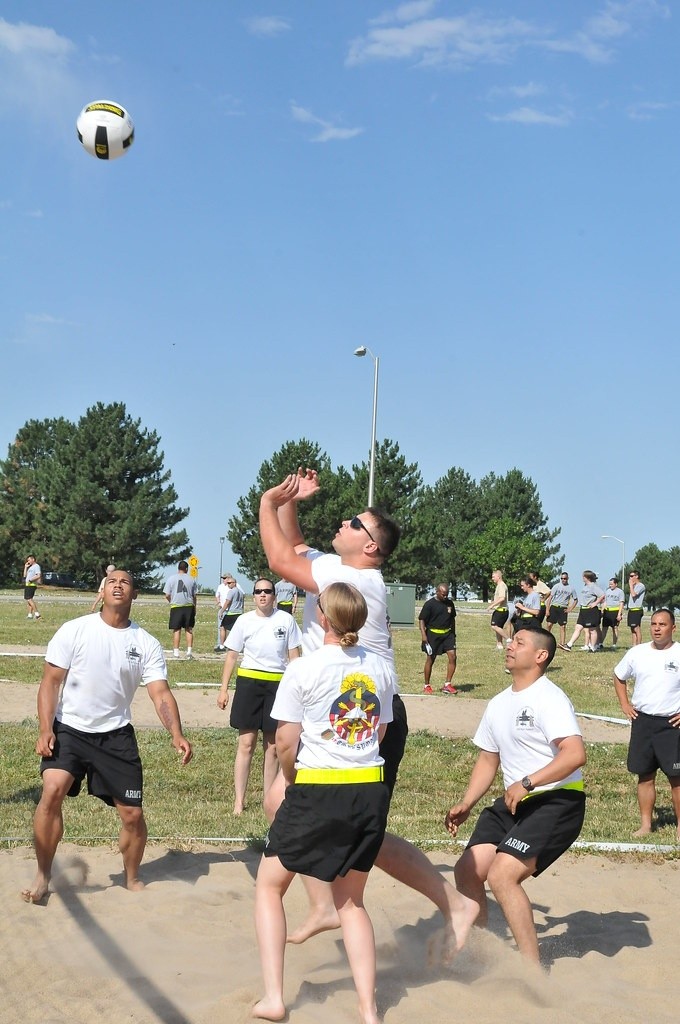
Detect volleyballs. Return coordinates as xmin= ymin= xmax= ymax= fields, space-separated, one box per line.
xmin=75 ymin=100 xmax=134 ymax=160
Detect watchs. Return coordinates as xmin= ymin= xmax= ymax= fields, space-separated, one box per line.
xmin=522 ymin=776 xmax=534 ymax=791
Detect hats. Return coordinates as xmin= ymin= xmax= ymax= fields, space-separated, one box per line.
xmin=221 ymin=573 xmax=231 ymax=578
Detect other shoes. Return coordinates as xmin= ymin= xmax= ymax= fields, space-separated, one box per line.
xmin=187 ymin=655 xmax=194 ymax=659
xmin=590 ymin=647 xmax=596 ymax=653
xmin=558 ymin=643 xmax=571 ymax=652
xmin=579 ymin=645 xmax=590 ymax=651
xmin=494 ymin=645 xmax=504 ymax=649
xmin=506 ymin=639 xmax=512 ymax=645
xmin=597 ymin=643 xmax=603 ymax=649
xmin=214 ymin=646 xmax=225 ymax=653
xmin=173 ymin=653 xmax=179 ymax=658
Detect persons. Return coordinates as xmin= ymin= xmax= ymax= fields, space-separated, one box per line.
xmin=274 ymin=578 xmax=298 ymax=615
xmin=163 ymin=561 xmax=200 ymax=657
xmin=258 ymin=467 xmax=480 ymax=961
xmin=24 ymin=555 xmax=41 ymax=620
xmin=217 ymin=578 xmax=302 ymax=815
xmin=252 ymin=582 xmax=394 ymax=1024
xmin=596 ymin=578 xmax=626 ymax=648
xmin=214 ymin=578 xmax=243 ymax=652
xmin=419 ymin=583 xmax=458 ymax=694
xmin=546 ymin=572 xmax=578 ymax=647
xmin=91 ymin=565 xmax=115 ymax=613
xmin=558 ymin=570 xmax=606 ymax=653
xmin=510 ymin=572 xmax=551 ymax=634
xmin=613 ymin=609 xmax=680 ymax=841
xmin=445 ymin=626 xmax=587 ymax=964
xmin=486 ymin=570 xmax=514 ymax=650
xmin=214 ymin=573 xmax=246 ymax=649
xmin=22 ymin=568 xmax=193 ymax=901
xmin=627 ymin=570 xmax=646 ymax=645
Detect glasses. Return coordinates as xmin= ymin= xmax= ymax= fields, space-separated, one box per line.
xmin=629 ymin=575 xmax=637 ymax=577
xmin=254 ymin=589 xmax=273 ymax=595
xmin=561 ymin=577 xmax=567 ymax=580
xmin=228 ymin=582 xmax=236 ymax=584
xmin=351 ymin=515 xmax=382 ymax=553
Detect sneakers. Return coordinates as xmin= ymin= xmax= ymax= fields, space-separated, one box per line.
xmin=442 ymin=683 xmax=458 ymax=694
xmin=424 ymin=686 xmax=433 ymax=694
